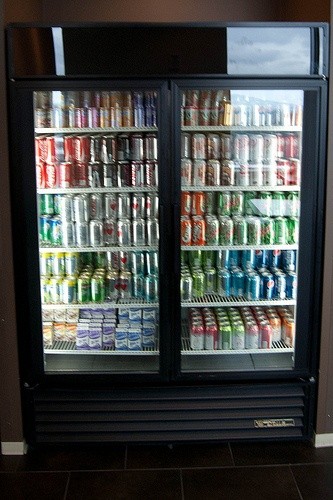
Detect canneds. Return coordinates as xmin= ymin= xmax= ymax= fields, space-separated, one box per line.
xmin=34 ymin=91 xmax=158 ymax=303
xmin=180 ymin=89 xmax=303 ymax=350
